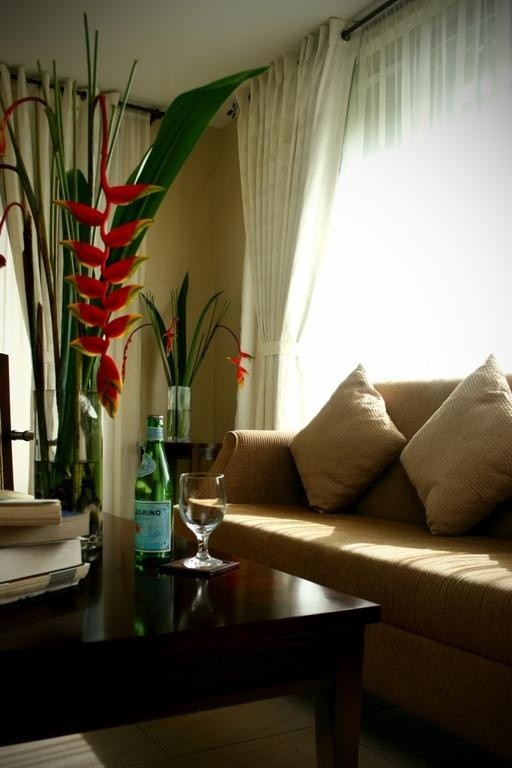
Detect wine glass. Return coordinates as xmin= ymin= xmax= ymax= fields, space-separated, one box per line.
xmin=179 ymin=577 xmax=226 ymax=631
xmin=179 ymin=472 xmax=228 ymax=570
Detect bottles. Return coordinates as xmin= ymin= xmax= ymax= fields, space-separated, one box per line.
xmin=132 ymin=574 xmax=175 ymax=634
xmin=133 ymin=415 xmax=176 ymax=570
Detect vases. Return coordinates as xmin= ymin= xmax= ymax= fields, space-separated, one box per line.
xmin=34 ymin=378 xmax=107 ymax=596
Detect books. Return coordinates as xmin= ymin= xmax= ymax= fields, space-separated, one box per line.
xmin=0 ymin=488 xmax=92 ymax=605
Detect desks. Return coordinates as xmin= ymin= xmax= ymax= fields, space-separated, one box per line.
xmin=138 ymin=441 xmax=223 ymax=505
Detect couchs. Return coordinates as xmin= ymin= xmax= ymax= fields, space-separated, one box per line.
xmin=173 ymin=374 xmax=512 ymax=755
